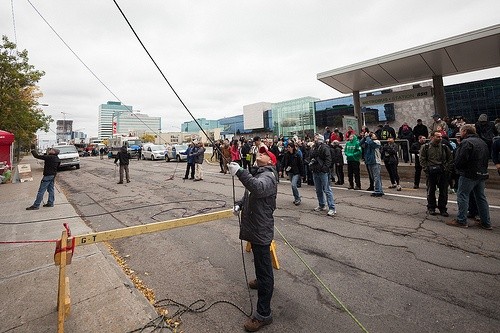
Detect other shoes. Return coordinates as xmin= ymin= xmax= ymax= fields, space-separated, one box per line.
xmin=314 ymin=205 xmax=326 ymax=211
xmin=474 ymin=219 xmax=492 ymax=230
xmin=332 ymin=178 xmax=421 ymax=197
xmin=117 ymin=182 xmax=123 ymax=184
xmin=293 ymin=198 xmax=301 ymax=205
xmin=183 ymin=177 xmax=204 ymax=181
xmin=445 ymin=218 xmax=468 ymax=228
xmin=43 ymin=203 xmax=53 ymax=207
xmin=328 ymin=209 xmax=336 ymax=216
xmin=429 ymin=208 xmax=449 ymax=217
xmin=467 ymin=211 xmax=479 ymax=218
xmin=127 ymin=180 xmax=130 ymax=183
xmin=26 ymin=206 xmax=39 ymax=210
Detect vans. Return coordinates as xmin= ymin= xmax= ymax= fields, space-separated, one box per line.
xmin=52 ymin=144 xmax=80 ymax=170
xmin=89 ymin=140 xmax=107 ymax=150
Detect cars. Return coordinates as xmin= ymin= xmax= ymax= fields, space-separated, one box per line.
xmin=165 ymin=144 xmax=189 ymax=163
xmin=140 ymin=145 xmax=168 ymax=161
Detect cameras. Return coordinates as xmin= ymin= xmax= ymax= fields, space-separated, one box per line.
xmin=365 ymin=125 xmax=372 ymax=137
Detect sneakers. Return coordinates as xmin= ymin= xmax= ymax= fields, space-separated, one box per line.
xmin=249 ymin=279 xmax=258 ymax=289
xmin=244 ymin=317 xmax=273 ymax=332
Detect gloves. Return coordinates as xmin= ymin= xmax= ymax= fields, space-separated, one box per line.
xmin=233 ymin=204 xmax=240 ymax=216
xmin=31 ymin=144 xmax=35 ymax=149
xmin=226 ymin=162 xmax=241 ymax=176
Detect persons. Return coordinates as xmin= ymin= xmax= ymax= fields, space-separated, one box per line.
xmin=179 ymin=141 xmax=205 ymax=181
xmin=210 ymin=136 xmax=269 ymax=174
xmin=408 ymin=112 xmax=500 ymax=230
xmin=307 ymin=132 xmax=337 ymax=215
xmin=99 ymin=147 xmax=106 ymax=160
xmin=281 ymin=143 xmax=302 ymax=205
xmin=25 ymin=144 xmax=61 ymax=210
xmin=323 ymin=124 xmax=356 ymax=142
xmin=263 ymin=132 xmax=344 ymax=188
xmin=163 ymin=142 xmax=173 ymax=162
xmin=226 ymin=146 xmax=278 ymax=333
xmin=113 ymin=145 xmax=131 ymax=184
xmin=374 ymin=123 xmax=414 ymax=163
xmin=359 ymin=131 xmax=383 ymax=193
xmin=136 ymin=146 xmax=142 ymax=161
xmin=343 ymin=134 xmax=362 ymax=190
xmin=357 ymin=125 xmax=369 ymax=140
xmin=381 ymin=137 xmax=401 ymax=191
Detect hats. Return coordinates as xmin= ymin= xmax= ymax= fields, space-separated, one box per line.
xmin=259 ymin=147 xmax=277 ymax=164
xmin=53 ymin=149 xmax=59 ymax=155
xmin=378 ymin=124 xmax=383 ymax=128
xmin=479 ymin=114 xmax=487 ymax=121
xmin=250 ymin=136 xmax=261 ymax=143
xmin=331 ymin=140 xmax=339 ymax=146
xmin=431 ymin=113 xmax=440 ymax=117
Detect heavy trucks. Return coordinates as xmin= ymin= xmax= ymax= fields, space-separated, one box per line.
xmin=109 ymin=137 xmax=143 ymax=159
